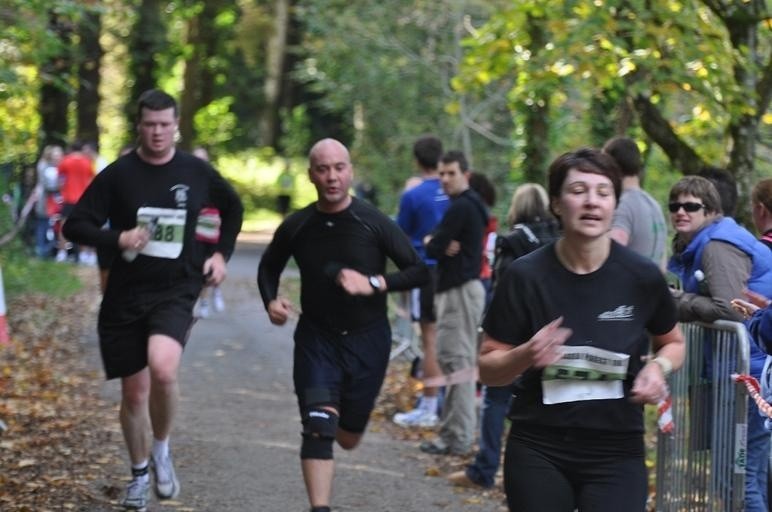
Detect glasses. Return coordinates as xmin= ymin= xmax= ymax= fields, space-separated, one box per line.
xmin=668 ymin=202 xmax=708 ymax=212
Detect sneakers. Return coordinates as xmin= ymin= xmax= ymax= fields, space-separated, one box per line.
xmin=446 ymin=470 xmax=484 ymax=489
xmin=419 ymin=439 xmax=450 ymax=454
xmin=124 ymin=475 xmax=151 ymax=511
xmin=392 ymin=409 xmax=439 ymax=428
xmin=151 ymin=446 xmax=180 ymax=499
xmin=213 ymin=287 xmax=227 ymax=314
xmin=200 ymin=300 xmax=213 ymax=320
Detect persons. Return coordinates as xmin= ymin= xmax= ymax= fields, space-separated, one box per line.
xmin=62 ymin=86 xmax=244 ymax=512
xmin=393 ymin=136 xmax=560 ymax=491
xmin=603 ymin=136 xmax=771 ymax=510
xmin=477 ymin=147 xmax=685 ymax=512
xmin=14 ymin=138 xmax=225 ymax=319
xmin=257 ymin=136 xmax=432 ymax=511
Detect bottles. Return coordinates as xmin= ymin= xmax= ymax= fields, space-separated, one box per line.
xmin=120 ymin=216 xmax=160 ymax=262
xmin=694 ymin=270 xmax=710 ymax=296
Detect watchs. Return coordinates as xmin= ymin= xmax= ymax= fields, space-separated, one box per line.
xmin=367 ymin=272 xmax=380 ymax=295
